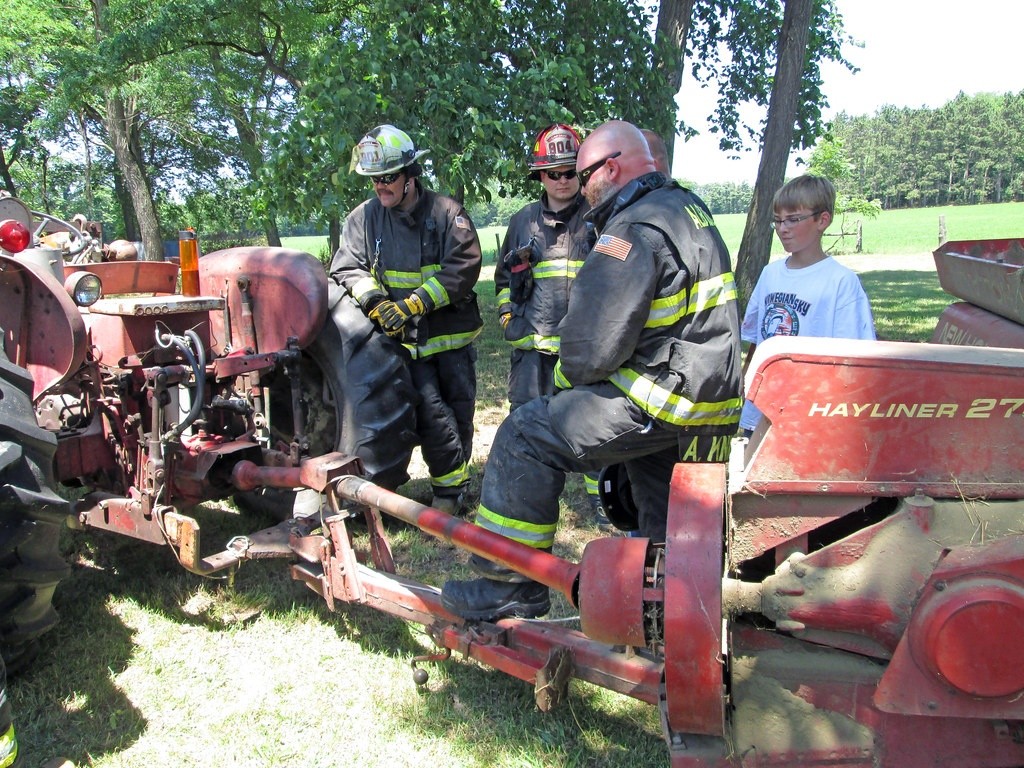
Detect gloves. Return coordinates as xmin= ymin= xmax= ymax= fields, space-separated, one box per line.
xmin=367 ymin=300 xmax=406 ymax=338
xmin=378 ymin=293 xmax=428 ymax=331
xmin=503 ymin=245 xmax=534 ymax=306
xmin=498 ymin=312 xmax=511 ymax=330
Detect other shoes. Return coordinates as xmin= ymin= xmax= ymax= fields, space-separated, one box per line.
xmin=588 ymin=492 xmax=613 ymax=526
xmin=418 ymin=492 xmax=464 ymax=538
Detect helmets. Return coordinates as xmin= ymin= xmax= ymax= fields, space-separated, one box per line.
xmin=526 ymin=122 xmax=582 ymax=171
xmin=347 ymin=124 xmax=432 ymax=176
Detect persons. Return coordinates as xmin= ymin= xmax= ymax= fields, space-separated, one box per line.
xmin=328 ymin=125 xmax=482 ymax=517
xmin=440 ymin=121 xmax=743 ymax=619
xmin=741 ymin=174 xmax=878 ymax=438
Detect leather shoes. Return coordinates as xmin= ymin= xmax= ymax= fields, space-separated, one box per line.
xmin=439 ymin=576 xmax=551 ymax=624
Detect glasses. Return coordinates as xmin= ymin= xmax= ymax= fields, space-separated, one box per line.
xmin=369 ymin=171 xmax=403 ymax=186
xmin=537 ymin=169 xmax=576 ymax=181
xmin=769 ymin=209 xmax=832 ymax=230
xmin=574 ymin=150 xmax=622 ymax=188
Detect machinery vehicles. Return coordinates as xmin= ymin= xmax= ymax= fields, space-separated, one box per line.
xmin=0 ymin=190 xmax=1023 ymax=768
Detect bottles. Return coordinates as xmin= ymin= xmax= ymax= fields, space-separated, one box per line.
xmin=179 ymin=230 xmax=201 ymax=297
